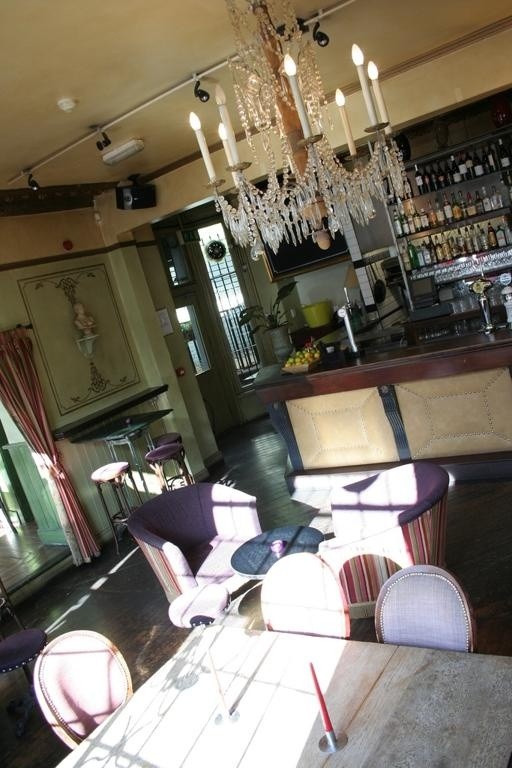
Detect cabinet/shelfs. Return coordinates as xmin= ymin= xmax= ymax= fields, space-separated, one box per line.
xmin=377 ymin=123 xmax=511 ymax=309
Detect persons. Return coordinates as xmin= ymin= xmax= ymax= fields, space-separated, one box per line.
xmin=73 ymin=303 xmax=97 ymax=336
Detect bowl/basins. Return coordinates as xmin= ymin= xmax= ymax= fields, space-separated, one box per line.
xmin=302 ymin=301 xmax=331 ymax=329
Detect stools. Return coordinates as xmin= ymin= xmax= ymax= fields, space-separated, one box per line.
xmin=148 ymin=432 xmax=183 ymax=451
xmin=90 ymin=461 xmax=146 ymax=556
xmin=145 ymin=444 xmax=193 ymax=491
xmin=1 ymin=626 xmax=49 ymax=684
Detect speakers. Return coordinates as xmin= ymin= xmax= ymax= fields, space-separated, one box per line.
xmin=115 ymin=183 xmax=157 ymax=210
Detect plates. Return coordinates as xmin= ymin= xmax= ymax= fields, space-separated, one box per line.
xmin=281 ymin=362 xmax=317 ymax=374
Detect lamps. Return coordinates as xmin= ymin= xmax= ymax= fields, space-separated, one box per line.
xmin=188 ymin=1 xmax=411 ymax=261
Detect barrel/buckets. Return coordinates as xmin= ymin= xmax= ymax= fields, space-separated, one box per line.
xmin=300 ymin=300 xmax=333 ymax=328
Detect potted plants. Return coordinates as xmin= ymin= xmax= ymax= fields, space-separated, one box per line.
xmin=238 ymin=281 xmax=298 ymax=364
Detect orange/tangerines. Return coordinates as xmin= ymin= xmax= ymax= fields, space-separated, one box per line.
xmin=284 ymin=349 xmax=320 ymax=368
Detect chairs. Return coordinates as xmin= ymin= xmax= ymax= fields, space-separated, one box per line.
xmin=38 ymin=629 xmax=134 ymax=750
xmin=373 ymin=563 xmax=473 ymax=654
xmin=322 ymin=461 xmax=458 ymax=630
xmin=259 ymin=549 xmax=352 ymax=640
xmin=124 ymin=480 xmax=263 ymax=615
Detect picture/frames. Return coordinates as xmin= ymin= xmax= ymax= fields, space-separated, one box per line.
xmin=261 ymin=232 xmax=354 ymax=283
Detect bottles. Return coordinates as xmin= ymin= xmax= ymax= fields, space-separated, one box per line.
xmin=384 ymin=136 xmax=512 ymax=273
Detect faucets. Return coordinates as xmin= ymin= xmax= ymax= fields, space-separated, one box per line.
xmin=338 ymin=302 xmax=358 ymax=354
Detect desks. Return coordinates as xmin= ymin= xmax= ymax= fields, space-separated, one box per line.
xmin=57 ymin=625 xmax=512 ymax=767
xmin=73 ymin=411 xmax=174 ymax=508
